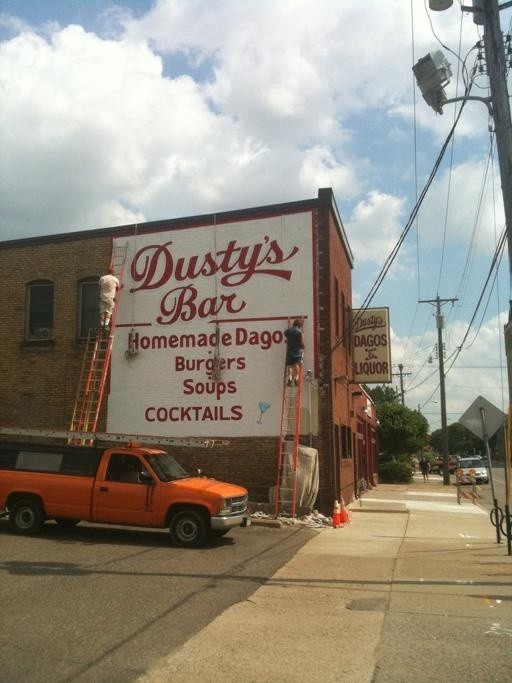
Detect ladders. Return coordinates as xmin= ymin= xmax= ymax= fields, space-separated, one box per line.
xmin=273 ymin=316 xmax=304 ymax=524
xmin=74 ymin=241 xmax=130 ymax=448
xmin=67 ymin=327 xmax=102 ymax=445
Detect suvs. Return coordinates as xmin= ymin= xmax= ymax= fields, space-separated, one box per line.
xmin=426 ymin=451 xmax=494 ymax=488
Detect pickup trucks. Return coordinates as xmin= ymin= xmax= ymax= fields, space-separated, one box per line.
xmin=0 ymin=439 xmax=252 ymax=548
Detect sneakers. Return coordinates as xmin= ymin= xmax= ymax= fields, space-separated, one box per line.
xmin=100 ymin=325 xmax=110 ymax=333
xmin=287 ymin=380 xmax=299 ymax=387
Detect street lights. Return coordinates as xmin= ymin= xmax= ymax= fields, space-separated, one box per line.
xmin=428 ymin=354 xmax=451 ymax=485
xmin=418 ymin=397 xmax=439 ymax=413
xmin=412 ymin=39 xmax=511 ymax=265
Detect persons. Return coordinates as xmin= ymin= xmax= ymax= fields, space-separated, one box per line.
xmin=97 ymin=266 xmax=120 ymax=330
xmin=419 ymin=457 xmax=430 ymax=483
xmin=284 ymin=320 xmax=305 ymax=386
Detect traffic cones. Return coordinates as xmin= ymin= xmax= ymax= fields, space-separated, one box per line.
xmin=340 ymin=495 xmax=351 ymax=522
xmin=329 ymin=498 xmax=344 ymax=528
